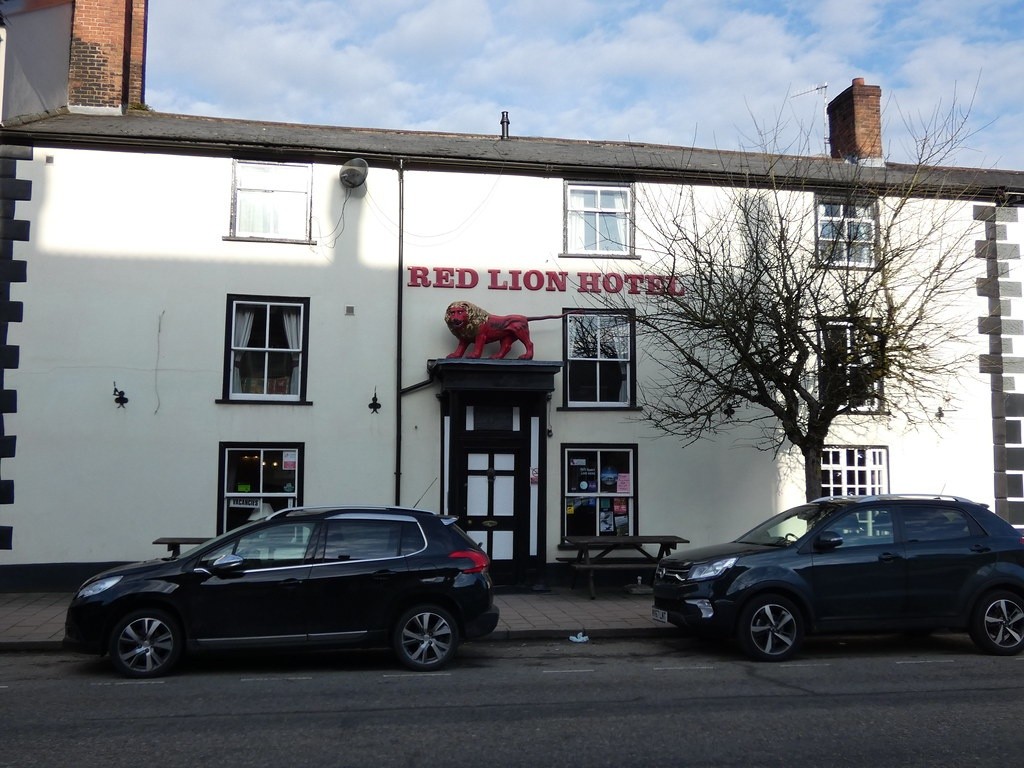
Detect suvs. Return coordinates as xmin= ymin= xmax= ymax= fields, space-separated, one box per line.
xmin=66 ymin=508 xmax=501 ymax=674
xmin=651 ymin=491 xmax=1024 ymax=657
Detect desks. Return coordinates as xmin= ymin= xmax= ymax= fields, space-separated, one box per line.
xmin=560 ymin=535 xmax=690 ymax=590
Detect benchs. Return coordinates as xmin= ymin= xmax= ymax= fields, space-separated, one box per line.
xmin=573 ymin=562 xmax=661 ymax=600
xmin=556 ymin=556 xmax=655 ymax=590
